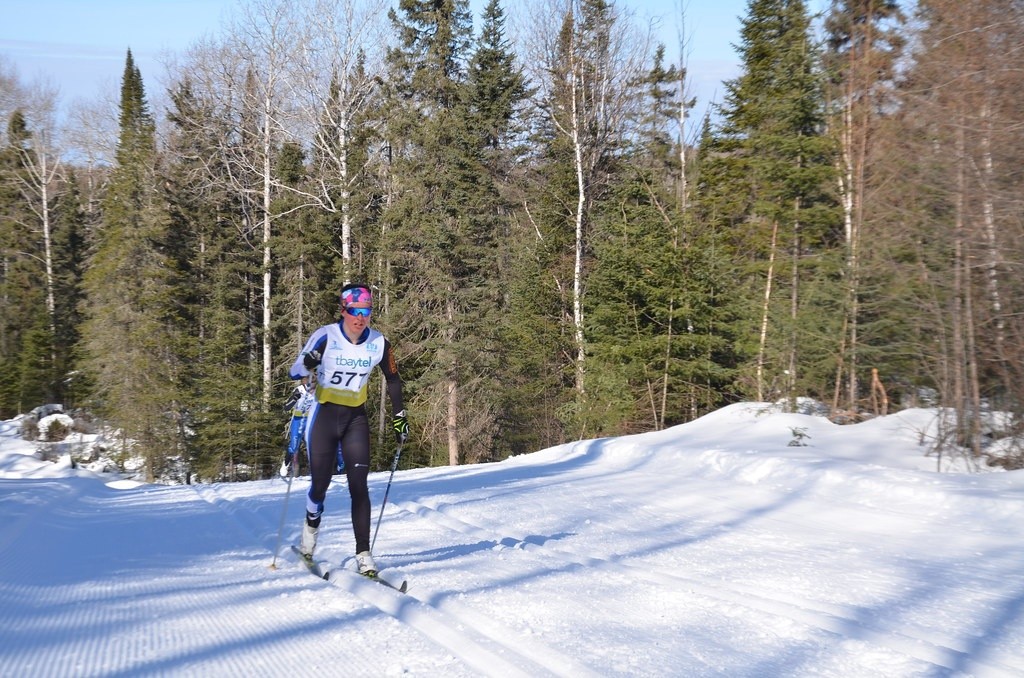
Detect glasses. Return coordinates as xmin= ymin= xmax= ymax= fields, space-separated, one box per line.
xmin=341 ymin=304 xmax=372 ymax=317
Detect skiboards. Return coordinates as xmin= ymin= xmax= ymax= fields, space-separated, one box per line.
xmin=291 ymin=543 xmax=408 ymax=594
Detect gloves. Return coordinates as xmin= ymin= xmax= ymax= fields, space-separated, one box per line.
xmin=304 ymin=350 xmax=322 ymax=369
xmin=392 ymin=417 xmax=409 ymax=445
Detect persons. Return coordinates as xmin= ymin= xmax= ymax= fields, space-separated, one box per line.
xmin=289 ymin=284 xmax=409 ymax=578
xmin=280 ymin=378 xmax=314 ymax=477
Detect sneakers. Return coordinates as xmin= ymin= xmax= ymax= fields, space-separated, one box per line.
xmin=302 ymin=522 xmax=317 ymax=558
xmin=280 ymin=460 xmax=291 ymax=477
xmin=356 ymin=551 xmax=378 ymax=577
